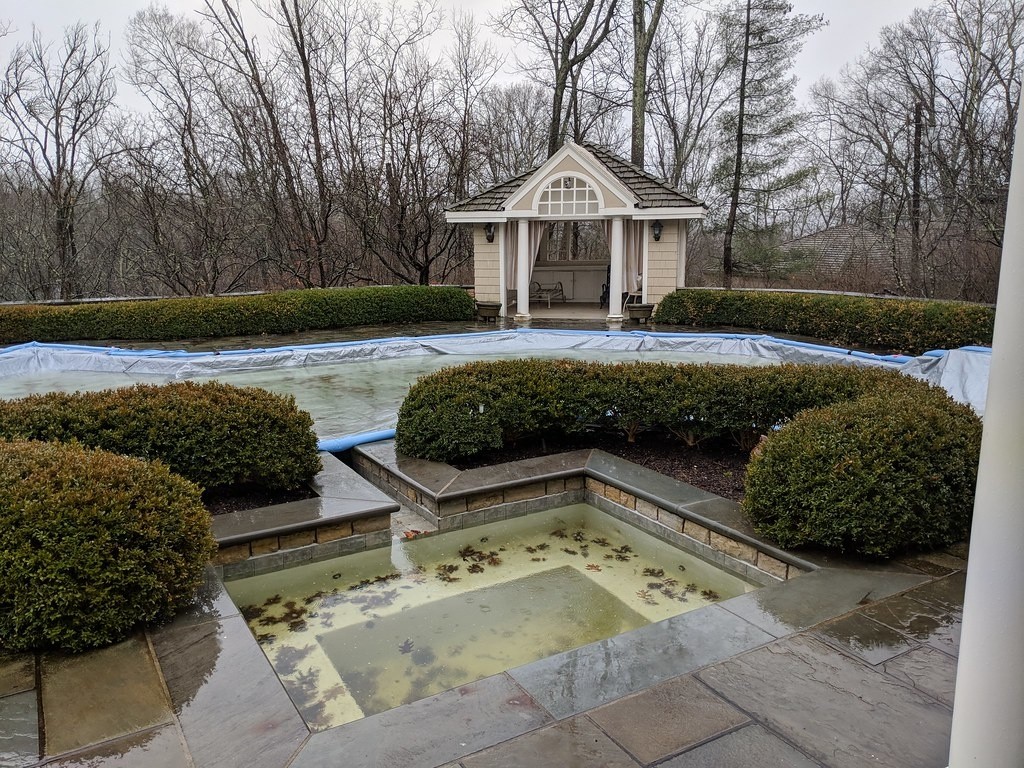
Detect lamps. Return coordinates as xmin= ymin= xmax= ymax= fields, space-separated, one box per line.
xmin=483 ymin=223 xmax=495 ymax=242
xmin=650 ymin=220 xmax=664 ymax=242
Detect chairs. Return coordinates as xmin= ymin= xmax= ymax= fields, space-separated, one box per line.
xmin=599 ymin=265 xmax=610 ymax=309
xmin=623 ymin=274 xmax=641 ymax=312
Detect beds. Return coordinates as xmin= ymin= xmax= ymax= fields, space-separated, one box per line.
xmin=529 ymin=281 xmax=565 ymax=309
